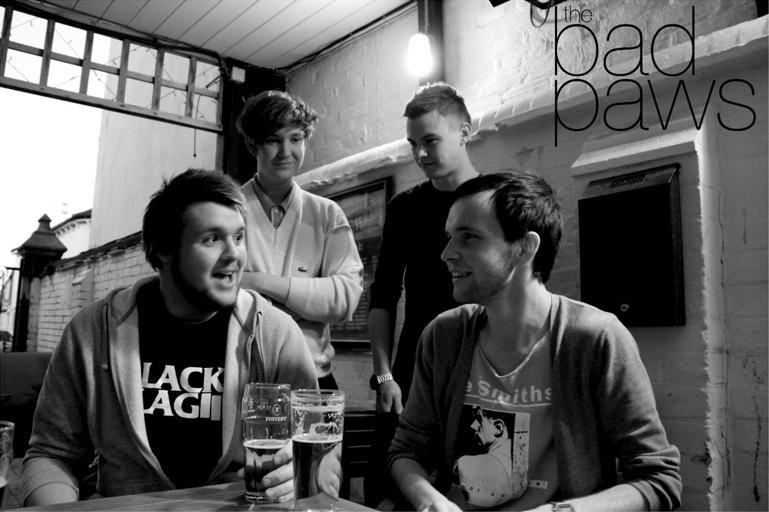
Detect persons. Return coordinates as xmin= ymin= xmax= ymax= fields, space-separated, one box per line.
xmin=386 ymin=172 xmax=684 ymax=512
xmin=20 ymin=169 xmax=322 ymax=507
xmin=362 ymin=81 xmax=482 ymax=510
xmin=298 ymin=445 xmax=344 ymax=501
xmin=236 ymin=437 xmax=297 ymax=504
xmin=451 ymin=406 xmax=513 ymax=507
xmin=237 ymin=89 xmax=366 ymax=502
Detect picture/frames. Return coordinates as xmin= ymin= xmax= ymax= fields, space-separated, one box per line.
xmin=0 ymin=480 xmax=382 ymax=512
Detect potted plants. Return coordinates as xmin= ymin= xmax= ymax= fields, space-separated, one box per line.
xmin=317 ymin=176 xmax=394 ymax=356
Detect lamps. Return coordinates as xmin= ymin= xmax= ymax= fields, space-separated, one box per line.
xmin=1 ymin=348 xmax=54 ymax=458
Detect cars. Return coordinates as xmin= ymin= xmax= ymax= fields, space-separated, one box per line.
xmin=291 ymin=389 xmax=344 ymax=510
xmin=1 ymin=421 xmax=14 ymax=512
xmin=241 ymin=384 xmax=291 ymax=505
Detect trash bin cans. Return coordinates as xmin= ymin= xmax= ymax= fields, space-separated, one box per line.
xmin=549 ymin=498 xmax=574 ymax=512
xmin=370 ymin=371 xmax=393 ymax=389
